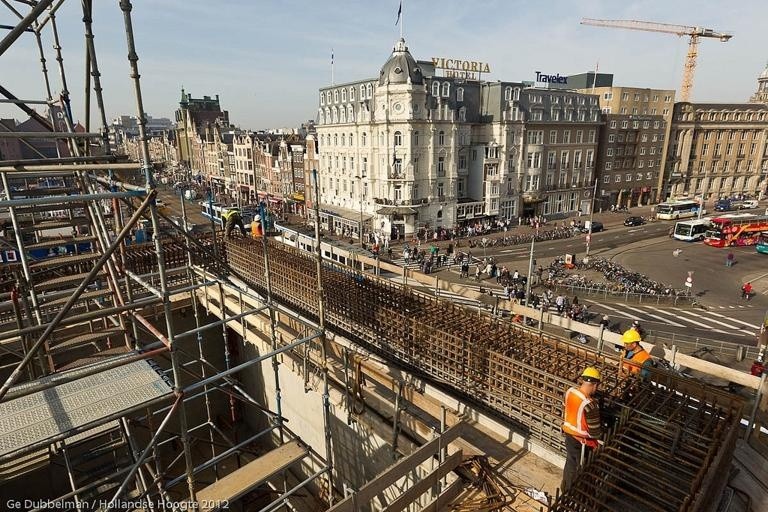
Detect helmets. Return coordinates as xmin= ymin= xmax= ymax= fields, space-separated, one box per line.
xmin=581 ymin=366 xmax=602 ymax=380
xmin=620 ymin=329 xmax=642 ymax=343
xmin=254 ymin=214 xmax=262 ymax=222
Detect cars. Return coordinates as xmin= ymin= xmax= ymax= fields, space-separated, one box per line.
xmin=624 ymin=216 xmax=643 ymax=226
xmin=592 ymin=222 xmax=603 ymax=232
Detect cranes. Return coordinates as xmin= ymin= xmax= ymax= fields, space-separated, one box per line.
xmin=580 ymin=15 xmax=733 ymax=101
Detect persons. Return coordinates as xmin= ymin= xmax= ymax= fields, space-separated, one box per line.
xmin=668 ymin=227 xmax=674 ymax=240
xmin=363 ymin=204 xmax=655 ymax=333
xmin=617 ymin=327 xmax=655 ymax=387
xmin=726 ymin=250 xmax=734 ymax=269
xmin=220 ymin=208 xmax=247 ymax=241
xmin=742 ymin=282 xmax=753 ymax=296
xmin=560 ymin=366 xmax=609 ymax=492
xmin=251 ymin=213 xmax=262 ymax=239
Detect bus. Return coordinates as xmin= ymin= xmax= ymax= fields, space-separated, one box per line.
xmin=202 ymin=201 xmax=252 ymax=231
xmin=656 ymin=198 xmax=768 ymax=253
